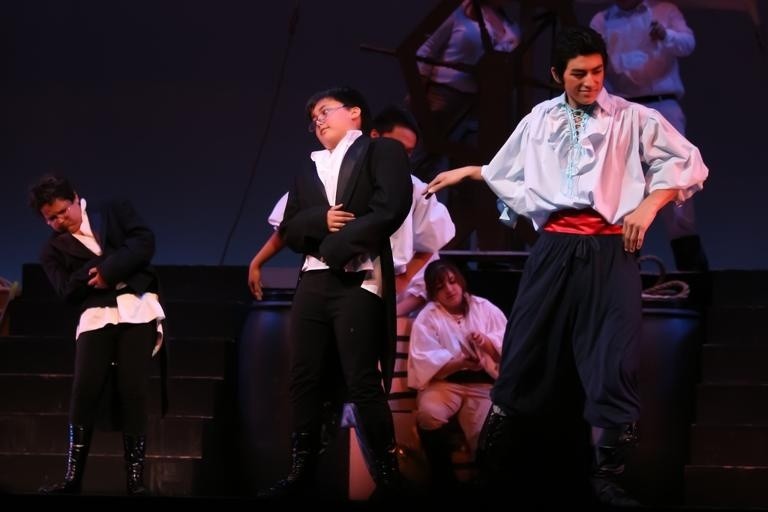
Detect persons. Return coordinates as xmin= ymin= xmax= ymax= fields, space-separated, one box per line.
xmin=27 ymin=174 xmax=167 ymax=497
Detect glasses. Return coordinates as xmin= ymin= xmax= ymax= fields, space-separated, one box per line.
xmin=307 ymin=103 xmax=347 ymax=134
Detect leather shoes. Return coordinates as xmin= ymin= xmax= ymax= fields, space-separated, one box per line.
xmin=624 ymin=93 xmax=677 ymax=104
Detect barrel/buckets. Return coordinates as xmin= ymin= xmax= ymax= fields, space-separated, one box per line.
xmin=234 ymin=301 xmax=292 ymax=448
xmin=637 ymin=309 xmax=702 ymax=432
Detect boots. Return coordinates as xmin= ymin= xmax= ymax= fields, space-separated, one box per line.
xmin=119 ymin=433 xmax=152 ymax=498
xmin=36 ymin=421 xmax=92 ymax=495
xmin=417 ymin=424 xmax=450 ymax=468
xmin=474 ymin=405 xmax=510 ymax=472
xmin=587 ymin=421 xmax=657 ymax=510
xmin=259 ymin=428 xmax=331 ymax=500
xmin=671 ymin=232 xmax=718 ymax=322
xmin=359 ymin=451 xmax=412 ymax=503
